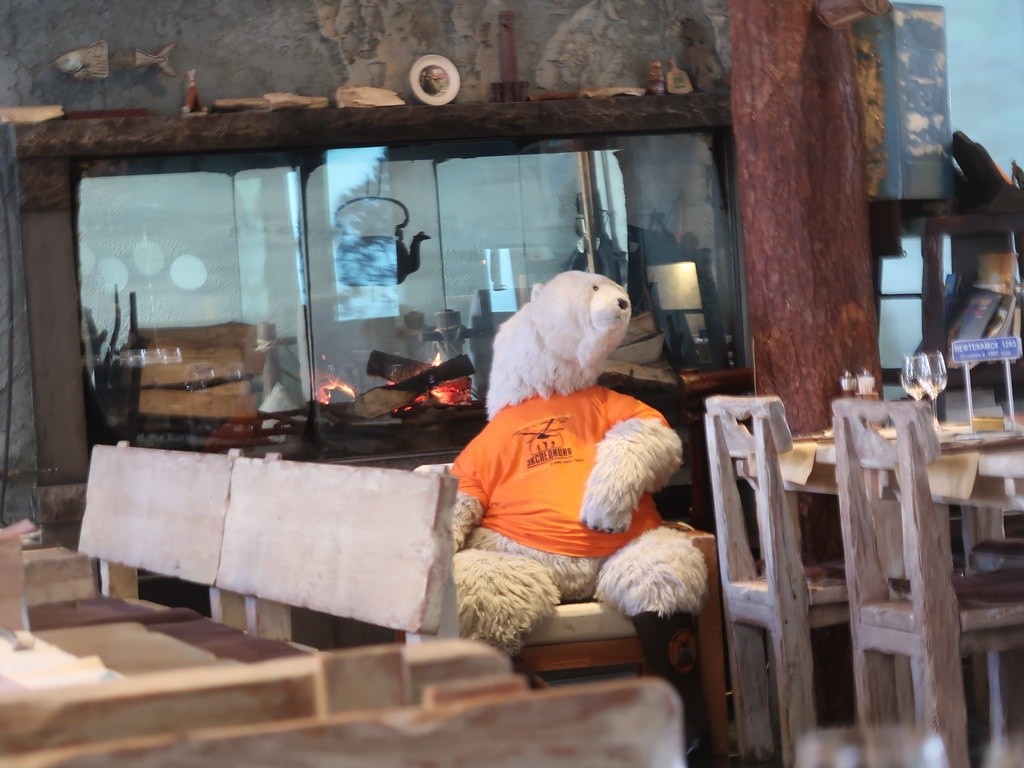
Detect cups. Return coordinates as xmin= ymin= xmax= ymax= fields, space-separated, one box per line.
xmin=901 ymin=357 xmax=931 ymax=400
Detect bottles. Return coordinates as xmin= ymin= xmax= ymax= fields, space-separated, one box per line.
xmin=650 ymin=61 xmax=664 ymax=94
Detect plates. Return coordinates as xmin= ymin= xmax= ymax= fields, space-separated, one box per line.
xmin=409 ymin=54 xmax=460 ymax=105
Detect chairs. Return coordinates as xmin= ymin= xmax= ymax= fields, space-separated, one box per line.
xmin=78 ymin=441 xmax=245 ymax=635
xmin=214 ymin=453 xmax=461 ymax=656
xmin=0 ymin=640 xmax=687 ymax=768
xmin=832 ymin=396 xmax=1023 ymax=768
xmin=405 ymin=462 xmax=731 ymax=755
xmin=704 ymin=394 xmax=851 ymax=768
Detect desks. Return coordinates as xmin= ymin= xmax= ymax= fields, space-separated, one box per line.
xmin=0 ymin=518 xmax=331 ymax=690
xmin=734 ymin=423 xmax=1024 ymax=578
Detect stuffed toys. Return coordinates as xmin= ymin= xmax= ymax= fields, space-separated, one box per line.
xmin=454 ymin=269 xmax=708 ymax=658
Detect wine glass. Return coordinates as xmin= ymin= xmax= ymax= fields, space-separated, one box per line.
xmin=918 ymin=351 xmax=948 ymax=432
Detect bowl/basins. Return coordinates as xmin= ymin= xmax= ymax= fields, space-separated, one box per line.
xmin=609 ymin=329 xmax=665 ymax=364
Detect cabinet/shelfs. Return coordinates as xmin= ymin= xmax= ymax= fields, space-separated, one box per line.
xmin=920 ymin=214 xmax=1024 ymax=425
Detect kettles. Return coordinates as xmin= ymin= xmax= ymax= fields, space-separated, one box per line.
xmin=334 ymin=197 xmax=432 ymax=286
